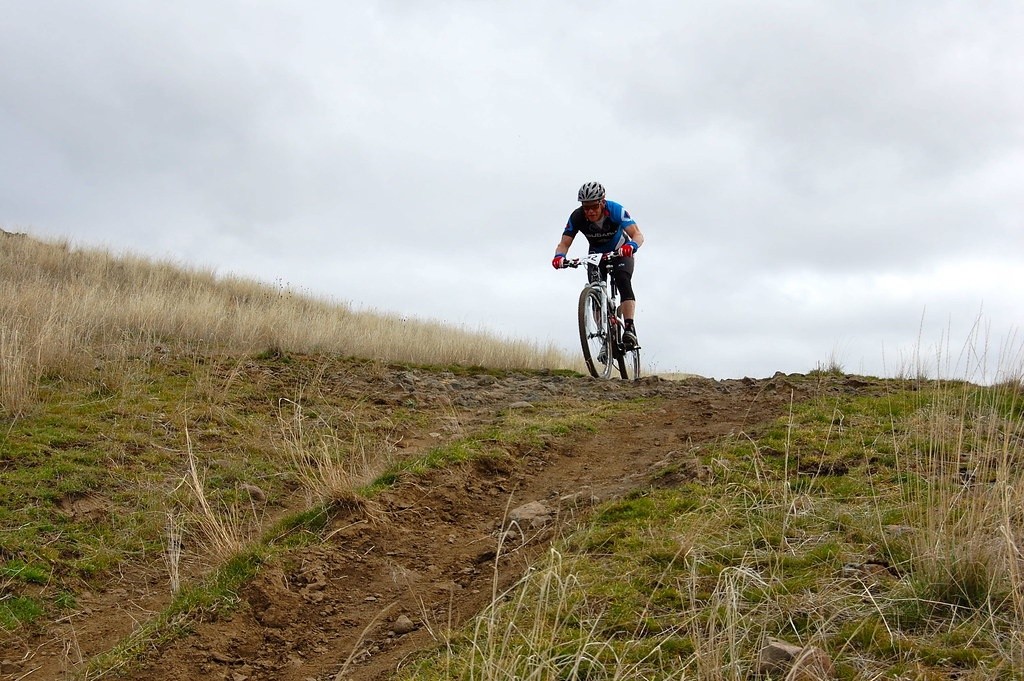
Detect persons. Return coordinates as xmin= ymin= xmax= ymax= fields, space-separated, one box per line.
xmin=552 ymin=182 xmax=644 ymax=361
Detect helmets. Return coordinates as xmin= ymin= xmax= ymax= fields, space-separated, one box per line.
xmin=578 ymin=182 xmax=605 ymax=201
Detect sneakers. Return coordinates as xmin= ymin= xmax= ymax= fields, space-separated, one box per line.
xmin=622 ymin=322 xmax=637 ymax=347
xmin=597 ymin=344 xmax=607 ymax=362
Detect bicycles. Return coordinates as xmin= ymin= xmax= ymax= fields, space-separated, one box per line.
xmin=563 ymin=248 xmax=641 ymax=380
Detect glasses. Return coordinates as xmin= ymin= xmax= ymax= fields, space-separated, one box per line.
xmin=582 ymin=200 xmax=600 ymax=210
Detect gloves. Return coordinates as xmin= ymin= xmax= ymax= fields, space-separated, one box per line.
xmin=618 ymin=244 xmax=634 ymax=258
xmin=552 ymin=254 xmax=566 ymax=269
